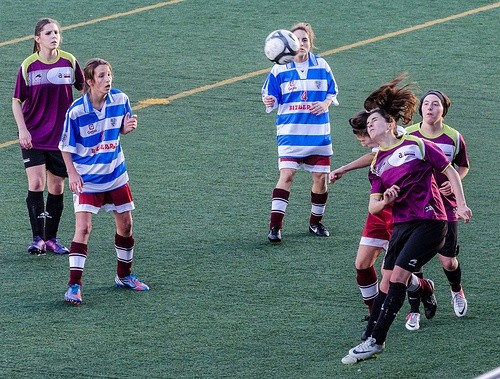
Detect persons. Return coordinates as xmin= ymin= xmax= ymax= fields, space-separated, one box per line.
xmin=58 ymin=57 xmax=150 ymax=305
xmin=327 ymin=72 xmax=469 ymax=365
xmin=11 ymin=18 xmax=87 ymax=254
xmin=261 ymin=23 xmax=338 ymax=242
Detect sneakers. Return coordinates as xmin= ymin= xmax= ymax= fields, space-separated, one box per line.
xmin=405 ymin=312 xmax=419 ymax=332
xmin=421 ymin=279 xmax=437 ymax=320
xmin=64 ymin=283 xmax=82 ymax=303
xmin=267 ymin=227 xmax=281 ymax=243
xmin=360 ymin=317 xmax=376 ymax=340
xmin=28 ymin=236 xmax=70 ymax=255
xmin=342 ymin=336 xmax=385 ymax=365
xmin=115 ymin=273 xmax=149 ymax=290
xmin=308 ymin=222 xmax=329 ymax=237
xmin=450 ymin=284 xmax=467 ymax=318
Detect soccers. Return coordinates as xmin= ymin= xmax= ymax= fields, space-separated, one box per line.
xmin=263 ymin=29 xmax=300 ymax=65
